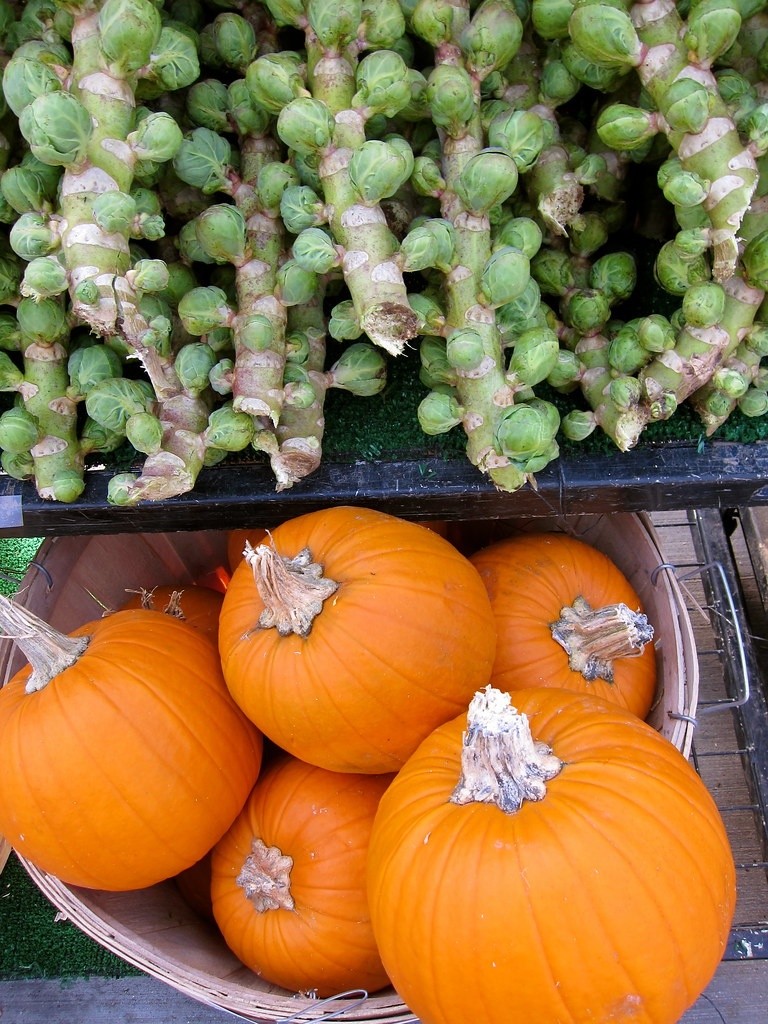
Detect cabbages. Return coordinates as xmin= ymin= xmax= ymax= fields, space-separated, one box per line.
xmin=0 ymin=0 xmax=768 ymax=506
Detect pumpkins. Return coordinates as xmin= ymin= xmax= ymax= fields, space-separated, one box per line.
xmin=0 ymin=506 xmax=738 ymax=1024
